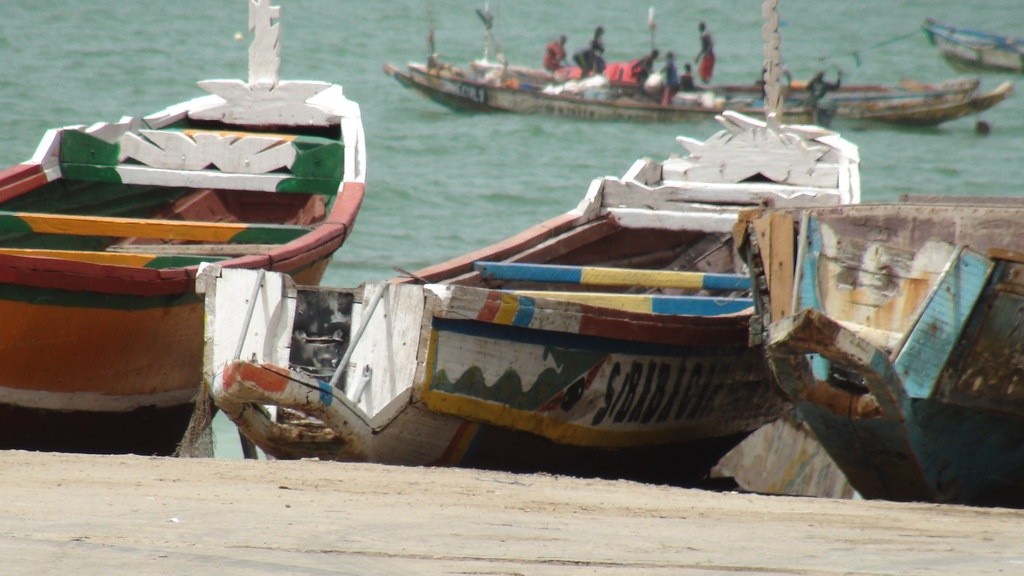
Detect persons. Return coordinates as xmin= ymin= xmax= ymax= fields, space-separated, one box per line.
xmin=543 ymin=19 xmax=716 ymax=106
xmin=796 ymin=72 xmax=840 ymax=110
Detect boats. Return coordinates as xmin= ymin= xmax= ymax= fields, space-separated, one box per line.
xmin=919 ymin=15 xmax=1024 ymax=70
xmin=384 ymin=0 xmax=1017 ymax=128
xmin=195 ymin=107 xmax=1024 ymax=510
xmin=0 ymin=0 xmax=366 ymax=457
